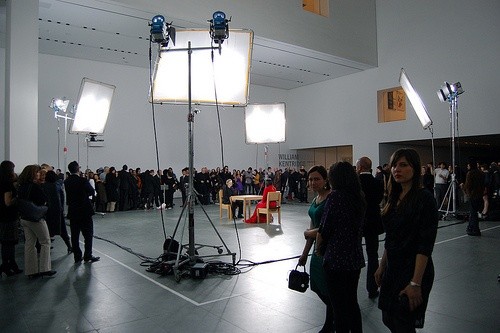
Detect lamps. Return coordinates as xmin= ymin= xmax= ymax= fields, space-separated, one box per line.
xmin=436 ymin=81 xmax=465 ymax=104
xmin=148 ymin=10 xmax=239 ymax=46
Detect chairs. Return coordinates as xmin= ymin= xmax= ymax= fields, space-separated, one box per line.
xmin=219 ymin=189 xmax=232 ymax=223
xmin=256 ymin=191 xmax=282 ymax=225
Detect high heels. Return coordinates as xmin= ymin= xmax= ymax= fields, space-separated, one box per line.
xmin=0 ymin=264 xmax=16 ymax=276
xmin=68 ymin=247 xmax=73 ymax=254
xmin=8 ymin=262 xmax=23 ymax=272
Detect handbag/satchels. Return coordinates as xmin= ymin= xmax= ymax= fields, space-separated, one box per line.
xmin=288 ymin=263 xmax=310 ymax=293
xmin=369 ymin=291 xmax=379 ymax=299
xmin=18 ymin=199 xmax=49 ymax=222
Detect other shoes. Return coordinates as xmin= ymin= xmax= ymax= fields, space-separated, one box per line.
xmin=238 ymin=215 xmax=244 ymax=218
xmin=84 ymin=256 xmax=100 ymax=263
xmin=75 ymin=259 xmax=81 ymax=266
xmin=40 ymin=271 xmax=56 ymax=276
xmin=467 ymin=230 xmax=481 ymax=236
xmin=232 ymin=216 xmax=237 ymax=219
xmin=30 ymin=274 xmax=40 ymax=279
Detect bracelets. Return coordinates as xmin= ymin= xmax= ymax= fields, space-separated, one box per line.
xmin=410 ymin=281 xmax=421 ymax=287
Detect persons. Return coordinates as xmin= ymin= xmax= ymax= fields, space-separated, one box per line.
xmin=373 ymin=148 xmax=439 ymax=333
xmin=0 ymin=160 xmax=500 ymax=281
xmin=289 ymin=157 xmax=383 ymax=333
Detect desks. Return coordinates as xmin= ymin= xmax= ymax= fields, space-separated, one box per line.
xmin=229 ymin=195 xmax=263 ymax=224
